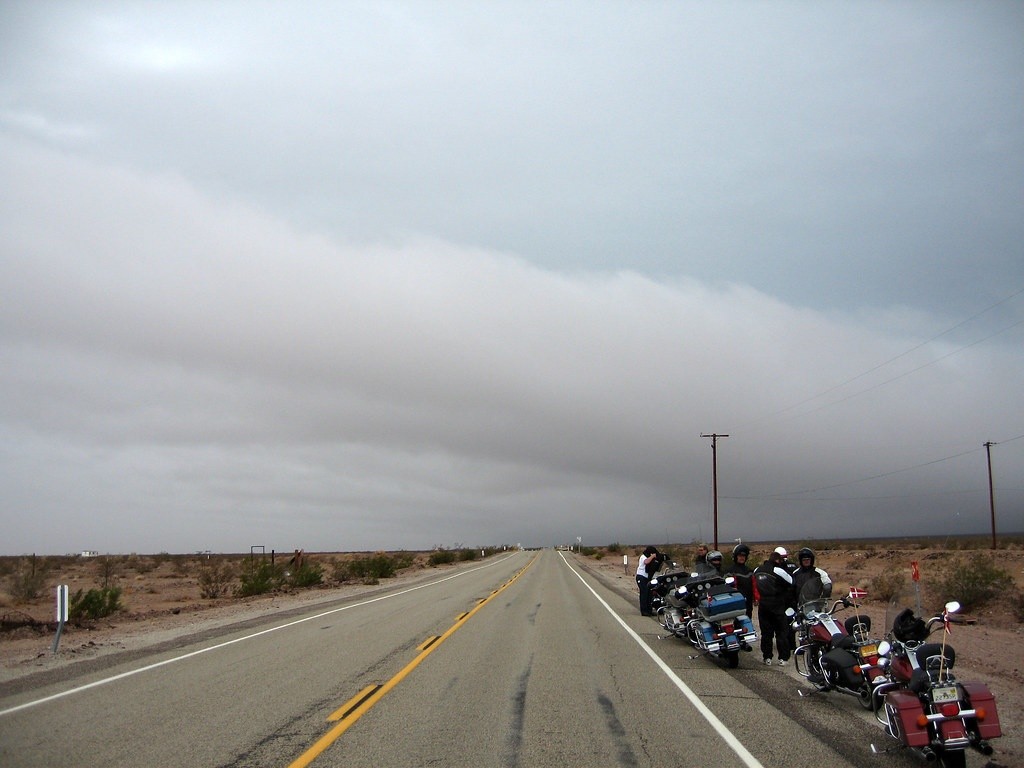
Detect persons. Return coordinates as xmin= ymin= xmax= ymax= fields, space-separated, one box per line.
xmin=635 ymin=547 xmax=661 ymax=615
xmin=696 ymin=543 xmax=832 ymax=668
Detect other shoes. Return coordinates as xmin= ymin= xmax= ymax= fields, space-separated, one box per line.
xmin=741 ymin=642 xmax=753 ymax=652
xmin=642 ymin=613 xmax=650 ymax=616
xmin=793 ymin=649 xmax=804 ymax=655
xmin=778 ymin=659 xmax=787 ymax=666
xmin=764 ymin=657 xmax=772 ymax=666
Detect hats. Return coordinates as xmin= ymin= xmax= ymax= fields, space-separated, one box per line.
xmin=774 ymin=546 xmax=788 ymax=560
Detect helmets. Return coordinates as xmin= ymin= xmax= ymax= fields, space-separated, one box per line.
xmin=706 ymin=550 xmax=724 ymax=568
xmin=894 ymin=608 xmax=931 ymax=641
xmin=799 ymin=547 xmax=816 ymax=565
xmin=731 ymin=544 xmax=749 ymax=563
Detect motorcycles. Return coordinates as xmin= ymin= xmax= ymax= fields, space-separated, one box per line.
xmin=643 ymin=557 xmax=759 ymax=670
xmin=871 ymin=558 xmax=1004 ymax=768
xmin=784 ymin=574 xmax=887 ymax=712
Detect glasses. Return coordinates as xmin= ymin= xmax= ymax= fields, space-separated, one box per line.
xmin=699 ymin=549 xmax=703 ymax=551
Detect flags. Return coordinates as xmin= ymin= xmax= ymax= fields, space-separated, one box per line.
xmin=912 ymin=560 xmax=920 ymax=582
xmin=850 ymin=585 xmax=866 ymax=598
xmin=943 ymin=610 xmax=952 ymax=636
